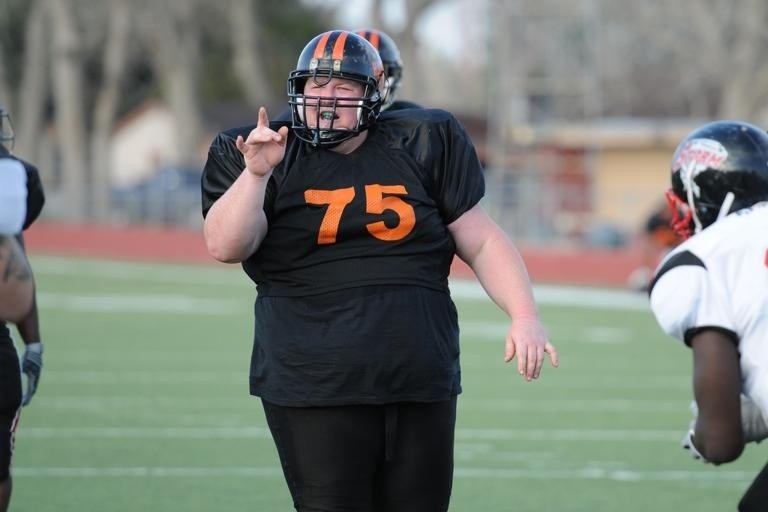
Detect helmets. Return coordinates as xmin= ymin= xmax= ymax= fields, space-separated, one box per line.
xmin=337 ymin=27 xmax=401 ymax=74
xmin=295 ymin=30 xmax=382 ymax=139
xmin=671 ymin=118 xmax=767 ymax=229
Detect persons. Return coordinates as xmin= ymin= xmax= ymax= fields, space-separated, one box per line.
xmin=648 ymin=120 xmax=768 ymax=512
xmin=270 ymin=30 xmax=424 ymax=123
xmin=200 ymin=31 xmax=557 ymax=512
xmin=0 ymin=108 xmax=44 ymax=512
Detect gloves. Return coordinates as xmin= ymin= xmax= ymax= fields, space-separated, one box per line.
xmin=21 ymin=343 xmax=44 ymax=404
